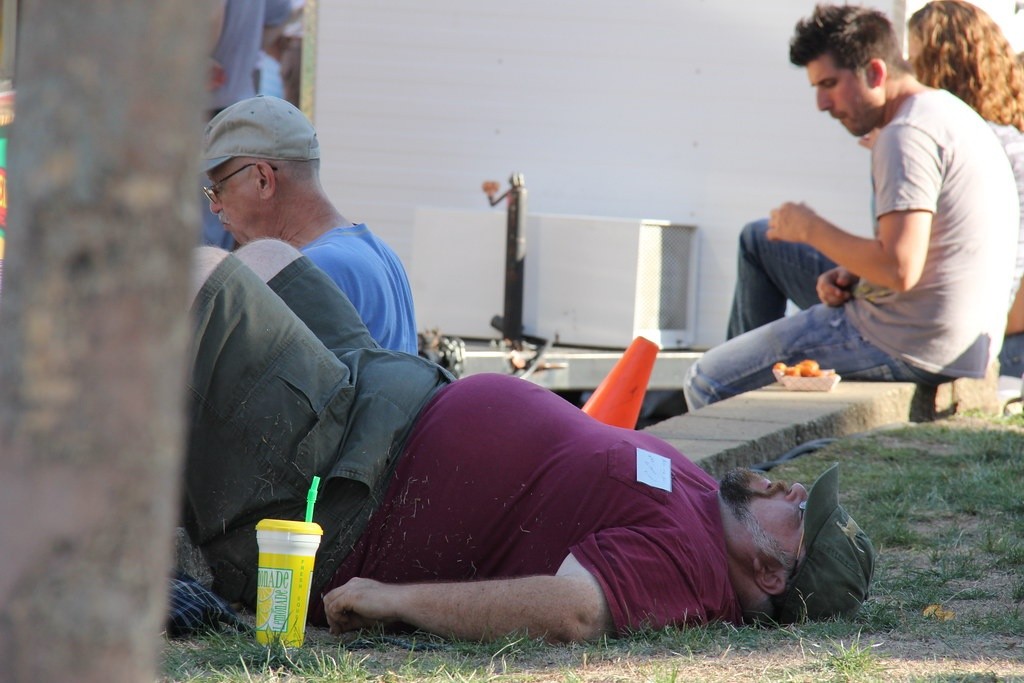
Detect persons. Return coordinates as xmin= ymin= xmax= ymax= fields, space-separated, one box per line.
xmin=187 ymin=240 xmax=876 ymax=648
xmin=683 ymin=3 xmax=1017 ymax=415
xmin=907 ymin=0 xmax=1023 ymax=381
xmin=206 ymin=1 xmax=307 ymax=252
xmin=204 ymin=94 xmax=417 ymax=355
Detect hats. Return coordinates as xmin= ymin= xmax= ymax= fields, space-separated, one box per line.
xmin=772 ymin=461 xmax=875 ymax=623
xmin=201 ymin=94 xmax=320 ymax=174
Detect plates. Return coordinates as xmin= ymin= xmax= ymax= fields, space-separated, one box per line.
xmin=773 ymin=369 xmax=836 ymax=382
xmin=781 ymin=374 xmax=841 ymax=390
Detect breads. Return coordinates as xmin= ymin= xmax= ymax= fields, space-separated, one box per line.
xmin=775 ymin=359 xmax=823 ymax=377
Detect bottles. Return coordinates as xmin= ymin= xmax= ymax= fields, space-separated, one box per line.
xmin=255 ymin=518 xmax=323 ymax=647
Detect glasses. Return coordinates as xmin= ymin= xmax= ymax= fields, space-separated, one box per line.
xmin=798 ymin=499 xmax=809 ymax=557
xmin=202 ymin=163 xmax=278 ymax=203
